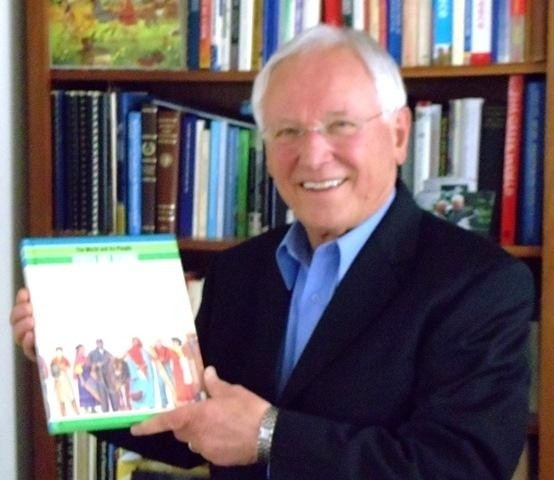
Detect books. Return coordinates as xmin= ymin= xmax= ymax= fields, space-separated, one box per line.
xmin=54 ymin=273 xmax=210 ymax=479
xmin=13 ymin=232 xmax=211 ymax=438
xmin=50 ymin=89 xmax=295 ymax=238
xmin=47 ymin=0 xmax=548 ymax=73
xmin=395 ymin=75 xmax=545 ymax=246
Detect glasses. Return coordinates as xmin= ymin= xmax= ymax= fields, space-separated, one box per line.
xmin=258 ymin=111 xmax=384 ymax=147
